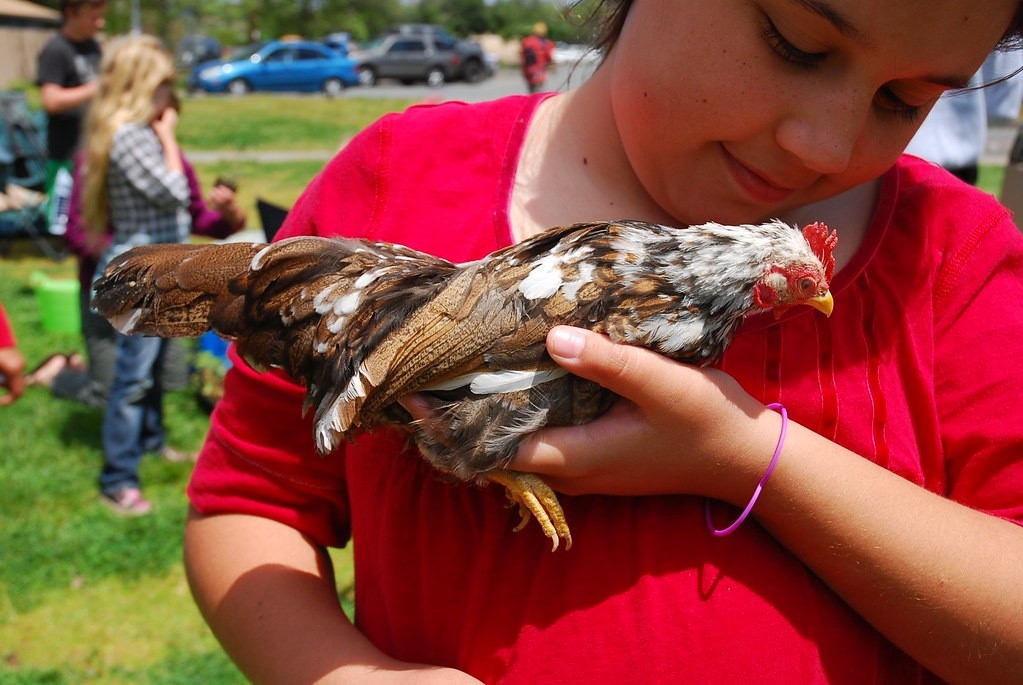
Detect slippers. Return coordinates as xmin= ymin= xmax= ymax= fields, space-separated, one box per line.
xmin=67 ymin=350 xmax=80 ymax=366
xmin=24 ymin=353 xmax=68 ymax=388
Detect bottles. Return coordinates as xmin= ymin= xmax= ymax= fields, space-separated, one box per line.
xmin=48 ymin=167 xmax=73 ymax=235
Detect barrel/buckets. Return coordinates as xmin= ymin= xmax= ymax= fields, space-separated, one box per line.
xmin=36 ymin=282 xmax=80 ymax=332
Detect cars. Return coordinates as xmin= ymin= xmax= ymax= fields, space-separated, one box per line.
xmin=188 ymin=39 xmax=360 ymax=97
xmin=391 ymin=25 xmax=499 ymax=86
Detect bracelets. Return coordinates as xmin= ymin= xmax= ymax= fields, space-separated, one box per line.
xmin=704 ymin=402 xmax=787 ymax=538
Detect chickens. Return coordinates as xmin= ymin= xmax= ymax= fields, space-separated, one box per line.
xmin=87 ymin=220 xmax=838 ymax=552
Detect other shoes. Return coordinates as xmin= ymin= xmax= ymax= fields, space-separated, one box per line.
xmin=101 ymin=485 xmax=151 ymax=516
xmin=161 ymin=446 xmax=184 ymax=463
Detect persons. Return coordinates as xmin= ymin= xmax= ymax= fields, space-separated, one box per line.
xmin=33 ymin=0 xmax=113 ymax=224
xmin=521 ymin=20 xmax=556 ymax=94
xmin=68 ymin=91 xmax=246 ymax=408
xmin=181 ymin=0 xmax=1023 ymax=685
xmin=74 ymin=30 xmax=192 ymax=518
xmin=900 ymin=31 xmax=1022 ymax=188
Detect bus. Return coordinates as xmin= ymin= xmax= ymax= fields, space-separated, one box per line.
xmin=349 ymin=22 xmax=459 ymax=86
